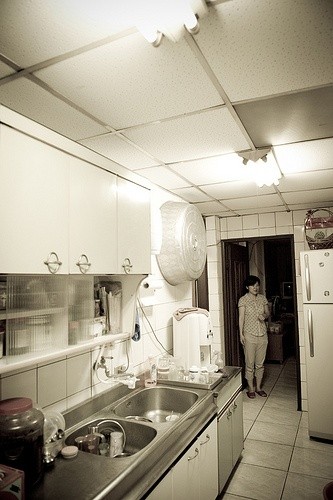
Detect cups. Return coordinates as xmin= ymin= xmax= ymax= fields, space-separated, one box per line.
xmin=158 ymin=355 xmax=211 ymax=384
xmin=75 ymin=432 xmax=124 ymax=457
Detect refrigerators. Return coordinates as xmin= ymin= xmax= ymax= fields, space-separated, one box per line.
xmin=299 ymin=248 xmax=333 ymax=441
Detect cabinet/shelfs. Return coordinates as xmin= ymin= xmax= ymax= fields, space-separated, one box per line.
xmin=0 ymin=119 xmax=151 ymax=277
xmin=145 ymin=390 xmax=245 ymax=500
xmin=265 ymin=329 xmax=290 ymax=364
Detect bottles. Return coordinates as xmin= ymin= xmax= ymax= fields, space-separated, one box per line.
xmin=143 ymin=354 xmax=157 ymax=387
xmin=0 ymin=397 xmax=45 ymax=491
xmin=94 ymin=299 xmax=100 ymax=317
xmin=0 ymin=281 xmax=56 ymax=360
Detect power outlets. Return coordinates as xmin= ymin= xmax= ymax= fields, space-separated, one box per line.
xmin=115 ymin=366 xmax=125 ymax=376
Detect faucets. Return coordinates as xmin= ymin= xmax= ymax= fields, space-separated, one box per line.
xmin=97 ymin=356 xmax=114 ymax=373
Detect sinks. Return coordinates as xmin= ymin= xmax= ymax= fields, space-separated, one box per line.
xmin=63 ymin=418 xmax=158 ymax=458
xmin=112 ymin=387 xmax=199 ymax=423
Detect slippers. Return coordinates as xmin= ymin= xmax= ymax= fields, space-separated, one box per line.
xmin=246 ymin=392 xmax=256 ymax=399
xmin=256 ymin=391 xmax=267 ymax=397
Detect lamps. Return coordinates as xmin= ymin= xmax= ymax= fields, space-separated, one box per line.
xmin=132 ymin=0 xmax=209 ymax=48
xmin=236 ymin=145 xmax=284 ymax=190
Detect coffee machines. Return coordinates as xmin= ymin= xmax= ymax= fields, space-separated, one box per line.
xmin=172 ymin=308 xmax=219 ymax=374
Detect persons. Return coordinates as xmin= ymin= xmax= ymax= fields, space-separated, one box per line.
xmin=238 ymin=275 xmax=271 ymax=399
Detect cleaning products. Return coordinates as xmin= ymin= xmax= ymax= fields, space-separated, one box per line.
xmin=145 ymin=354 xmax=157 ymax=388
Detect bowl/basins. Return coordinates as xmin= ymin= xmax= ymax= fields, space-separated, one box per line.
xmin=97 ymin=420 xmax=127 ymax=450
xmin=33 ymin=404 xmax=66 ymax=457
